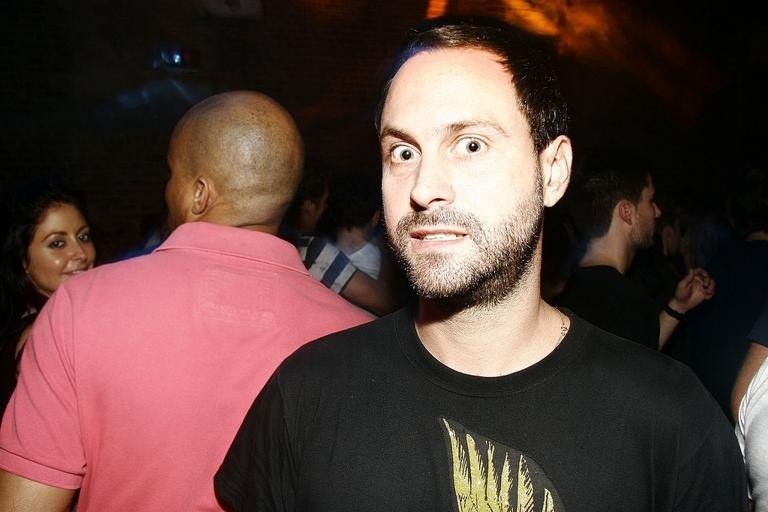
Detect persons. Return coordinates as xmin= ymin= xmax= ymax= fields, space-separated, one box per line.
xmin=1 ymin=87 xmax=381 ymax=512
xmin=3 ymin=146 xmax=767 ymax=512
xmin=212 ymin=16 xmax=749 ymax=509
